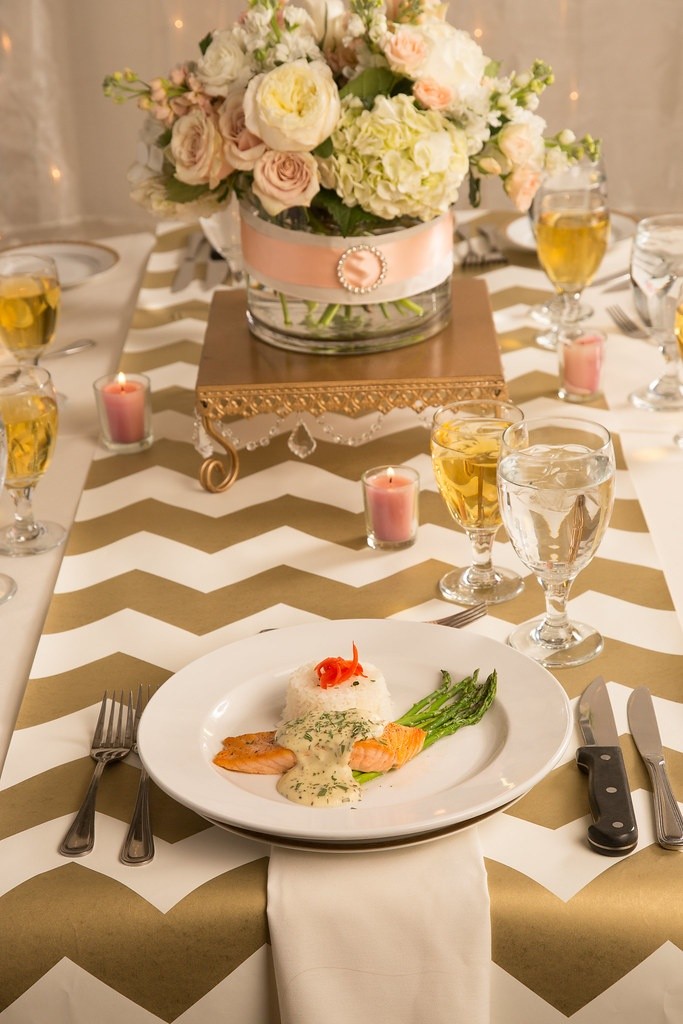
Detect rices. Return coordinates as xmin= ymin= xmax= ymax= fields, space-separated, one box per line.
xmin=277 ymin=661 xmax=392 ymax=728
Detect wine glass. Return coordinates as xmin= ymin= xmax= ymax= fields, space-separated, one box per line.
xmin=0 ymin=365 xmax=67 ymax=558
xmin=530 ymin=160 xmax=606 ymax=321
xmin=498 ymin=416 xmax=616 ymax=667
xmin=534 ymin=192 xmax=608 ymax=350
xmin=0 ymin=251 xmax=66 ymax=406
xmin=430 ymin=400 xmax=528 ymax=606
xmin=628 ymin=214 xmax=683 ymax=411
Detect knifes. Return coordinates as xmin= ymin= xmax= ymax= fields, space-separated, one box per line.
xmin=171 ymin=232 xmax=228 ymax=292
xmin=574 ymin=676 xmax=639 ymax=854
xmin=625 ymin=684 xmax=682 ymax=850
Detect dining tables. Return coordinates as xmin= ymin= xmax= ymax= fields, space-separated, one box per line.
xmin=0 ymin=215 xmax=683 ymax=1024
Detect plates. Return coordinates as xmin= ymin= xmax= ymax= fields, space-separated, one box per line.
xmin=198 ymin=790 xmax=529 ymax=854
xmin=505 ymin=213 xmax=634 ymax=252
xmin=0 ymin=238 xmax=119 ymax=289
xmin=135 ymin=619 xmax=573 ymax=841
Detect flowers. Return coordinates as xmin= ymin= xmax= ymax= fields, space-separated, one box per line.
xmin=101 ymin=0 xmax=602 ymax=331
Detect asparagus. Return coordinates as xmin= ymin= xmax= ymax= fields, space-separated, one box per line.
xmin=349 ymin=668 xmax=498 ymax=782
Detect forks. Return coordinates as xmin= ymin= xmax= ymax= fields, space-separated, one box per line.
xmin=259 ymin=606 xmax=488 ymax=636
xmin=607 ymin=303 xmax=668 ymax=355
xmin=118 ymin=686 xmax=161 ymax=866
xmin=456 ymin=220 xmax=512 ymax=268
xmin=59 ymin=689 xmax=135 ymax=856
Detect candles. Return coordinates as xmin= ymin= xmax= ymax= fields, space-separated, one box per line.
xmin=103 ymin=372 xmax=146 ymax=443
xmin=564 ymin=333 xmax=603 ymax=393
xmin=367 ymin=468 xmax=415 ymax=542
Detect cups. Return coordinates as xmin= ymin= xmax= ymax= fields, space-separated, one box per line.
xmin=361 ymin=464 xmax=423 ymax=549
xmin=93 ymin=371 xmax=156 ymax=452
xmin=557 ymin=327 xmax=607 ymax=404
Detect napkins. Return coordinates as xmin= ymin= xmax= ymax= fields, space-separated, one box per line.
xmin=265 ymin=827 xmax=492 ymax=1024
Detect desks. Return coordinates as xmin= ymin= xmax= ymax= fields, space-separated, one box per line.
xmin=194 ymin=279 xmax=506 ymax=493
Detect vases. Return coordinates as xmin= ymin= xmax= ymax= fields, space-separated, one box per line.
xmin=238 ymin=201 xmax=454 ymax=355
